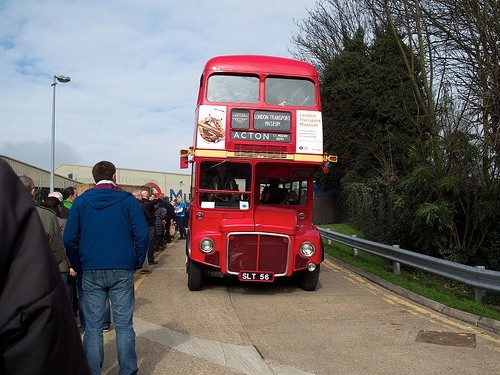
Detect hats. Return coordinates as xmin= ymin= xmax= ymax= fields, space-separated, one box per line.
xmin=47 ymin=192 xmax=63 ymax=202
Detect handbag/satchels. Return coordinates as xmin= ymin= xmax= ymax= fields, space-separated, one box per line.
xmin=169 ymin=220 xmax=176 ymax=238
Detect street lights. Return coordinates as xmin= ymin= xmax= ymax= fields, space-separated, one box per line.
xmin=50 ymin=73 xmax=71 ymax=193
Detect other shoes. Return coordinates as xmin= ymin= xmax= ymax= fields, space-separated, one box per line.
xmin=183 ymin=236 xmax=187 ymax=239
xmin=141 ymin=270 xmax=152 ymax=274
xmin=103 ymin=326 xmax=111 ymax=332
xmin=155 ymin=236 xmax=174 ymax=252
xmin=178 ymin=236 xmax=183 ymax=240
xmin=75 ymin=317 xmax=81 ymax=328
xmin=148 ymin=261 xmax=158 ymax=265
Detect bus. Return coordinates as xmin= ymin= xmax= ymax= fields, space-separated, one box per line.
xmin=180 ymin=55 xmax=338 ymax=291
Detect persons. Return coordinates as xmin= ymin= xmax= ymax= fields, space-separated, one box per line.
xmin=262 ymin=180 xmax=289 ymax=206
xmin=63 ymin=161 xmax=148 ymax=375
xmin=16 ymin=176 xmax=191 ymax=333
xmin=206 ymin=161 xmax=241 ymax=202
